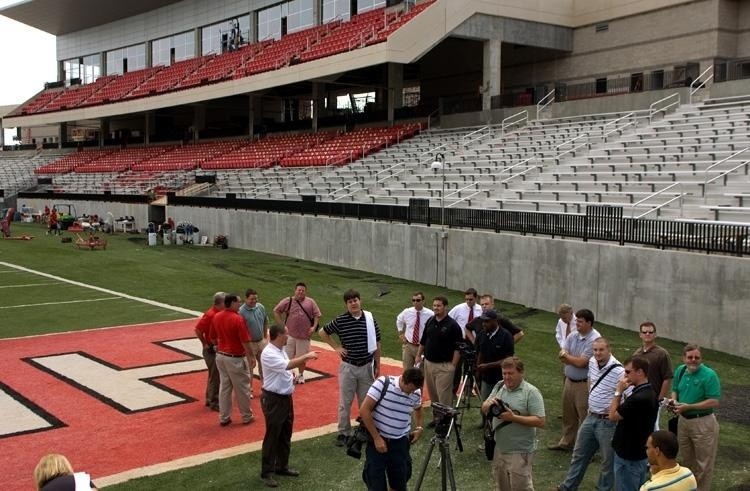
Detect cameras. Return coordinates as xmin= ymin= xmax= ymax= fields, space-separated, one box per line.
xmin=335 ymin=417 xmax=381 ymax=461
xmin=489 ymin=400 xmax=510 ymax=420
xmin=429 ymin=401 xmax=464 ymax=452
xmin=455 ymin=340 xmax=478 ymax=366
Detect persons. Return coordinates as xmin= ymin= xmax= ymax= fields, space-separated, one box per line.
xmin=272 ymin=282 xmax=322 ymax=384
xmin=607 ymin=354 xmax=658 ymax=491
xmin=30 ymin=452 xmax=98 ymax=491
xmin=415 ymin=297 xmax=463 ymax=429
xmin=638 ymin=430 xmax=697 ymax=491
xmin=464 ymin=295 xmax=524 ymax=347
xmin=481 ymin=356 xmax=545 ymax=489
xmin=448 ymin=288 xmax=483 ymax=399
xmin=630 ymin=320 xmax=673 ymax=431
xmin=20 ymin=204 xmax=31 ymax=214
xmin=548 ymin=309 xmax=602 ymax=453
xmin=396 ymin=292 xmax=435 ymax=373
xmin=259 ymin=324 xmax=321 ymax=488
xmin=237 ymin=288 xmax=262 ymax=397
xmin=556 ymin=336 xmax=636 ymax=491
xmin=195 ymin=292 xmax=229 ymax=413
xmin=472 ymin=309 xmax=514 ymax=461
xmin=669 ymin=342 xmax=722 ymax=490
xmin=45 ymin=208 xmax=63 ymax=237
xmin=555 ymin=303 xmax=578 ymax=350
xmin=318 ymin=289 xmax=383 ymax=447
xmin=207 ymin=292 xmax=259 ymax=419
xmin=358 ymin=366 xmax=425 ymax=490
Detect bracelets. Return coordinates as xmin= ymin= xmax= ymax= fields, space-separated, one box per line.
xmin=415 ymin=425 xmax=424 ymax=433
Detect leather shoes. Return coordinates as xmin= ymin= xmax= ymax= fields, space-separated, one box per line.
xmin=275 ymin=467 xmax=300 ymax=477
xmin=259 ymin=475 xmax=278 ymax=488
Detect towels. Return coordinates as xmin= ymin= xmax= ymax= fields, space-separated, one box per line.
xmin=363 ymin=309 xmax=377 ymax=355
xmin=73 ymin=471 xmax=92 ymax=491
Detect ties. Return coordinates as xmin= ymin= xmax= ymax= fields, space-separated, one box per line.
xmin=412 ymin=311 xmax=421 ymax=345
xmin=468 ymin=306 xmax=474 ymax=322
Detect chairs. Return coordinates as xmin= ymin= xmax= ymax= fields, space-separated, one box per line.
xmin=20 ymin=1 xmax=434 ymax=119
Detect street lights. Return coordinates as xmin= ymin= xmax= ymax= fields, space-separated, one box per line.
xmin=432 ymin=152 xmax=447 ymax=239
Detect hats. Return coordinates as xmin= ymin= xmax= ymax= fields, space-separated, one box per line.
xmin=479 ymin=308 xmax=498 ymax=321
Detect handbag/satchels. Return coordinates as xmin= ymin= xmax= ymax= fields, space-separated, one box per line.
xmin=668 ymin=365 xmax=687 ymax=436
xmin=296 ymin=298 xmax=320 ymax=332
xmin=483 ymin=380 xmax=505 ymax=461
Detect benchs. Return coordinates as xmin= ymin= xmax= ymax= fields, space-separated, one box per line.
xmin=2 ymin=94 xmax=750 ymax=255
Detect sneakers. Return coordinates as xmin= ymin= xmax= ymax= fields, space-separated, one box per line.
xmin=548 ymin=444 xmax=562 ymax=451
xmin=243 ymin=413 xmax=254 ymax=424
xmin=220 ymin=417 xmax=232 ymax=425
xmin=298 ymin=374 xmax=305 ymax=384
xmin=336 ymin=434 xmax=348 ymax=447
xmin=425 ymin=420 xmax=436 ymax=429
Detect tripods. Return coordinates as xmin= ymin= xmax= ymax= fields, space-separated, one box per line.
xmin=437 ymin=371 xmax=492 ymax=468
xmin=410 ymin=435 xmax=457 ymax=491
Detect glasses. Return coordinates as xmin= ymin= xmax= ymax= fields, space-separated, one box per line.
xmin=687 ymin=355 xmax=700 ymax=359
xmin=624 ymin=369 xmax=635 ymax=374
xmin=641 ymin=330 xmax=654 ymax=334
xmin=412 ymin=298 xmax=421 ymax=302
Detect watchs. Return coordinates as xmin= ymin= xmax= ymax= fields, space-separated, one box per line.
xmin=613 ymin=390 xmax=622 ymax=396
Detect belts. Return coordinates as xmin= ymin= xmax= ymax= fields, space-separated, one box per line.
xmin=341 ymin=357 xmax=373 ymax=367
xmin=565 ymin=322 xmax=570 ymax=339
xmin=382 ymin=436 xmax=406 ymax=443
xmin=588 ymin=410 xmax=609 ymax=419
xmin=217 ymin=352 xmax=246 ymax=358
xmin=680 ymin=412 xmax=709 ymax=419
xmin=568 ymin=377 xmax=588 ymax=383
